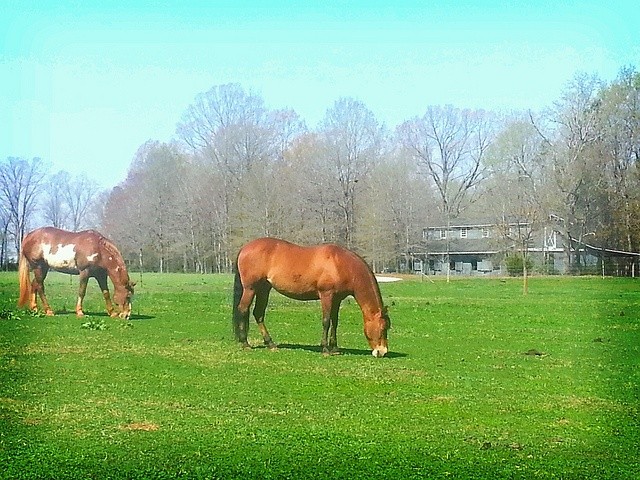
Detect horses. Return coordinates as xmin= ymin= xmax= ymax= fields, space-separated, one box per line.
xmin=231 ymin=237 xmax=393 ymax=359
xmin=16 ymin=225 xmax=139 ymax=321
